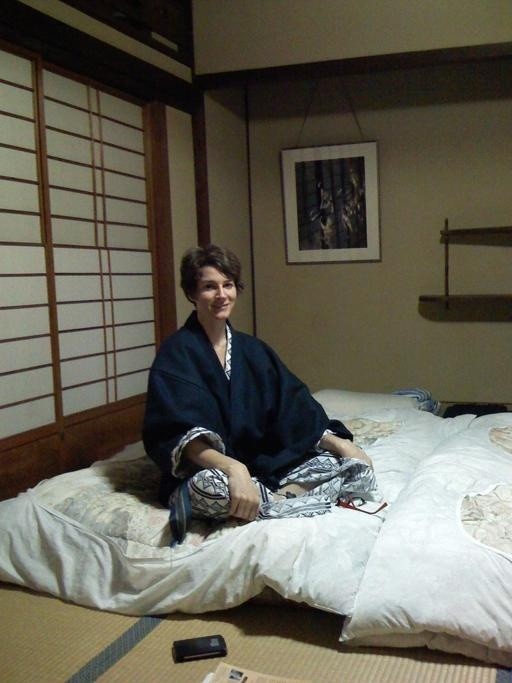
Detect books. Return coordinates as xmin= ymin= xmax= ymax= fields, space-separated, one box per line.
xmin=202 ymin=661 xmax=304 ymax=683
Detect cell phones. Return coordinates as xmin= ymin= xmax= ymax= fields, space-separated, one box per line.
xmin=173 ymin=635 xmax=227 ymax=663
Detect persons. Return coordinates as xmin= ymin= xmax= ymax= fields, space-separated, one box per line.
xmin=142 ymin=244 xmax=383 ymax=529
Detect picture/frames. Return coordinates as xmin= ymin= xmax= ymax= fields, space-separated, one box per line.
xmin=277 ymin=138 xmax=382 ymax=266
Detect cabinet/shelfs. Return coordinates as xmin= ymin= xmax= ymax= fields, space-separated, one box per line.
xmin=416 ymin=216 xmax=512 ymax=312
